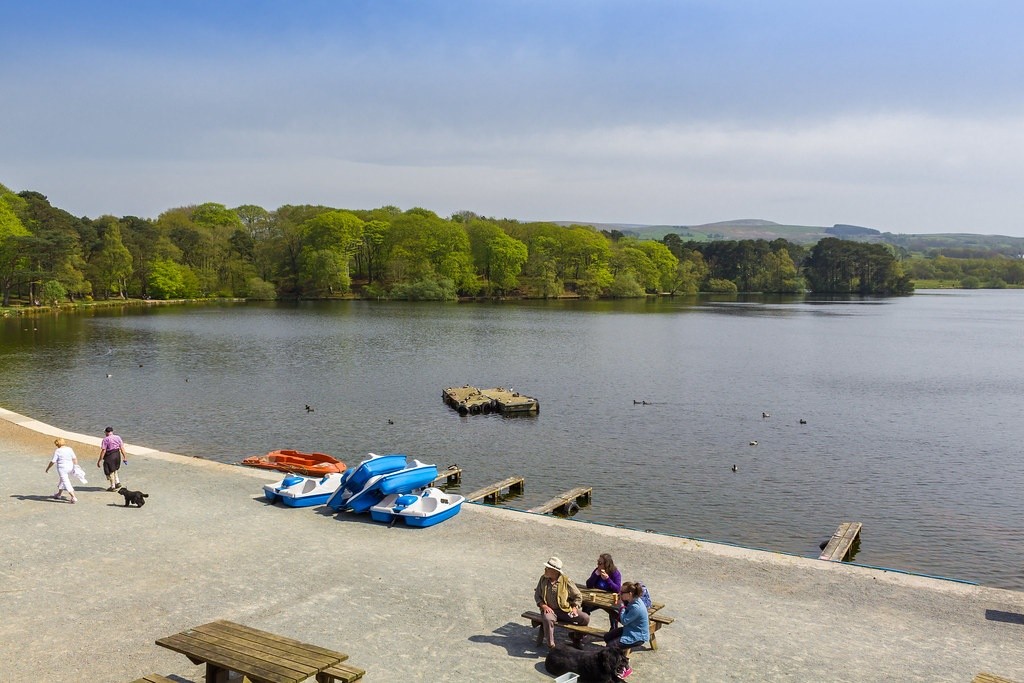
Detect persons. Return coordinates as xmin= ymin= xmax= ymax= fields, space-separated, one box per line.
xmin=583 ymin=554 xmax=621 ymax=630
xmin=535 ymin=557 xmax=590 ymax=653
xmin=604 ymin=582 xmax=649 ymax=678
xmin=98 ymin=426 xmax=126 ymax=491
xmin=46 ymin=438 xmax=77 ymax=503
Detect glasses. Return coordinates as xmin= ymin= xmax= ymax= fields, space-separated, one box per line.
xmin=597 ymin=560 xmax=605 ymax=565
xmin=620 ymin=591 xmax=629 ymax=595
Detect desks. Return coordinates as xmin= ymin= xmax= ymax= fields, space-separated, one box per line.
xmin=533 ymin=582 xmax=666 ymax=651
xmin=155 ymin=619 xmax=349 ymax=683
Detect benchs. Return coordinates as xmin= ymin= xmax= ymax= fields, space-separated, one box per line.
xmin=650 ymin=615 xmax=674 ymax=624
xmin=130 ymin=662 xmax=366 ymax=683
xmin=522 ymin=610 xmax=631 ymax=639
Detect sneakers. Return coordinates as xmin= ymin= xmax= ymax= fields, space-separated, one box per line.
xmin=617 ymin=667 xmax=633 ymax=680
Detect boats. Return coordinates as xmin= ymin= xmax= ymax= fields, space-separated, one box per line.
xmin=241 ymin=449 xmax=347 ymax=476
xmin=369 ymin=486 xmax=464 ymax=528
xmin=342 ymin=458 xmax=439 ymax=514
xmin=325 ymin=451 xmax=407 ymax=512
xmin=262 ymin=473 xmax=346 ymax=507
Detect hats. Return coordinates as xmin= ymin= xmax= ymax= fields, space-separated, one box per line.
xmin=543 ymin=557 xmax=564 ymax=575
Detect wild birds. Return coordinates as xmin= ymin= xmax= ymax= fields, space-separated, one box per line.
xmin=632 ymin=399 xmax=807 ymax=474
xmin=305 ymin=404 xmax=394 ymax=426
xmin=105 ymin=364 xmax=190 ymax=383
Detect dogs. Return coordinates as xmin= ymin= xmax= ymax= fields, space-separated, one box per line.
xmin=119 ymin=488 xmax=149 ymax=508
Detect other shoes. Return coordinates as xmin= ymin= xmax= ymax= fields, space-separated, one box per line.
xmin=55 ymin=494 xmax=61 ymax=499
xmin=69 ymin=497 xmax=78 ymax=503
xmin=116 ymin=483 xmax=121 ymax=488
xmin=106 ymin=487 xmax=116 ymax=491
xmin=568 ymin=632 xmax=584 ymax=649
